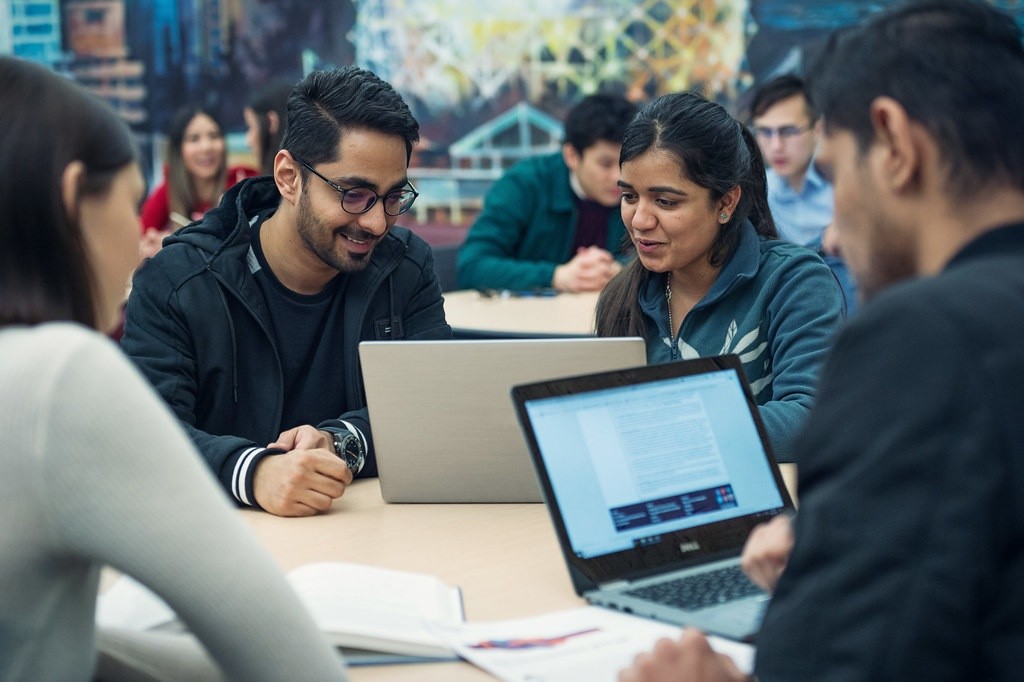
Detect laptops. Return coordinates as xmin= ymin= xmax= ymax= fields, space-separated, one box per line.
xmin=358 ymin=338 xmax=646 ymax=504
xmin=509 ymin=352 xmax=799 ymax=648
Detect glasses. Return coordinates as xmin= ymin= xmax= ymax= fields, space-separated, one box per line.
xmin=290 ymin=151 xmax=419 ymax=216
xmin=748 ymin=120 xmax=817 ymax=141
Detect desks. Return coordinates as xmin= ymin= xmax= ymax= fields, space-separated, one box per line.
xmin=442 ymin=287 xmax=610 ymax=335
xmin=97 ymin=462 xmax=802 ymax=682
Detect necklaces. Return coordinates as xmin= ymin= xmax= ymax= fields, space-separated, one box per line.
xmin=665 ymin=271 xmax=674 ymax=344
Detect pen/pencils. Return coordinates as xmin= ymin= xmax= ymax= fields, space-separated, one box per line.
xmin=169 ymin=210 xmax=193 ymax=227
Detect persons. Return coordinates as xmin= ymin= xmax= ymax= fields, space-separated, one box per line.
xmin=119 ymin=64 xmax=452 ymax=518
xmin=0 ymin=0 xmax=1024 ymax=682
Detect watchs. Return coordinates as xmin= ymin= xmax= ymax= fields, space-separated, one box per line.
xmin=316 ymin=426 xmax=363 ymax=474
